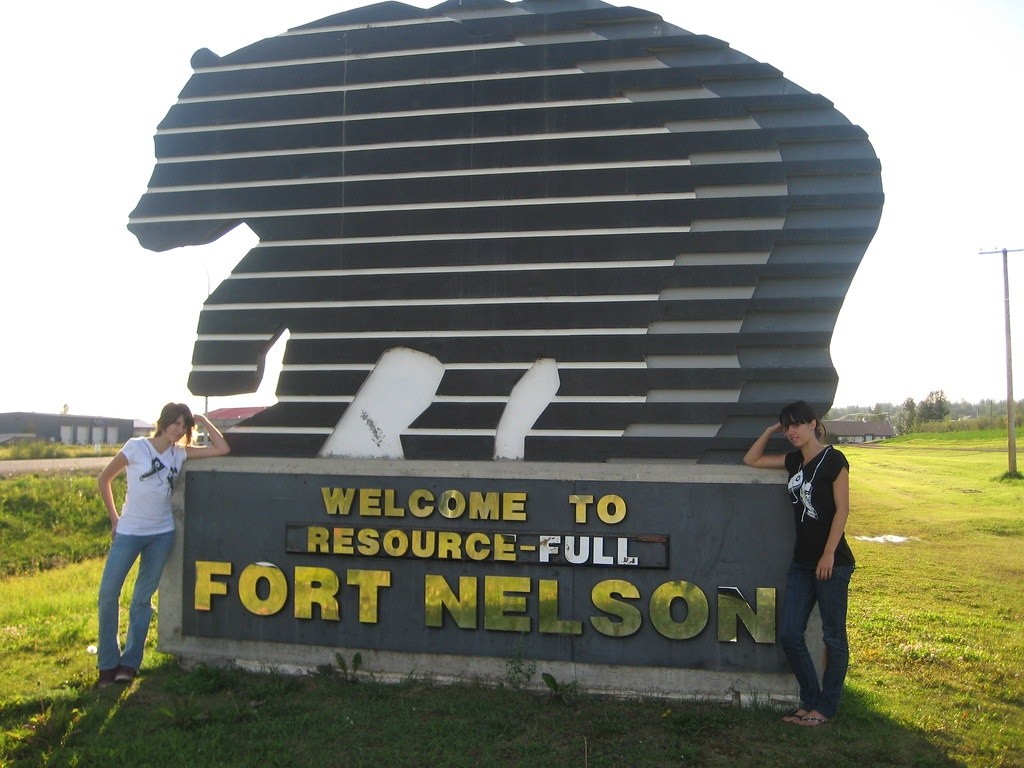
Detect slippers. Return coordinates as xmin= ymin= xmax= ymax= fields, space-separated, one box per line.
xmin=802 ymin=716 xmax=826 ymax=724
xmin=783 ymin=713 xmax=802 ymax=725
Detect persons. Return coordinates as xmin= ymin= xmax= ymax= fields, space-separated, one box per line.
xmin=743 ymin=400 xmax=856 ymax=727
xmin=94 ymin=402 xmax=232 ymax=689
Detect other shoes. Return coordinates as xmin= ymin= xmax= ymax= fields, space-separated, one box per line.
xmin=115 ymin=666 xmax=134 ymax=682
xmin=98 ymin=670 xmax=117 ymax=688
